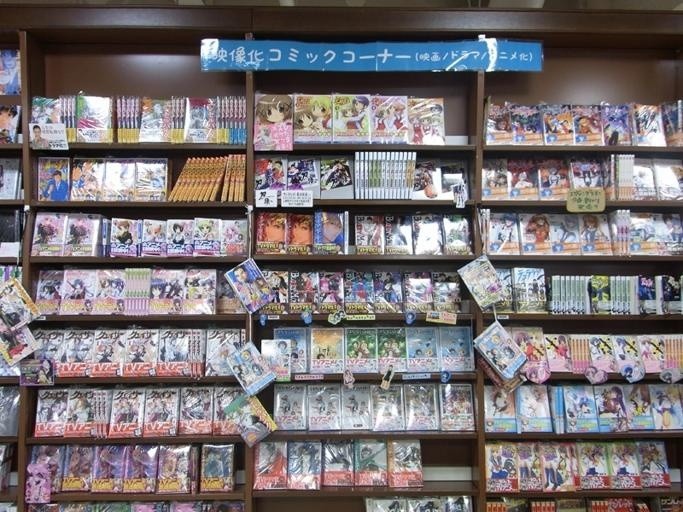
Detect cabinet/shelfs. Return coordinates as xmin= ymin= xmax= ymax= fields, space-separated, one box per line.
xmin=0 ymin=1 xmax=683 ymax=511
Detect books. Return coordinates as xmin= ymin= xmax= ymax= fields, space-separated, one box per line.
xmin=227 ymin=90 xmax=477 ymax=511
xmin=0 ymin=51 xmax=248 ymax=511
xmin=460 ymin=101 xmax=682 ymax=511
